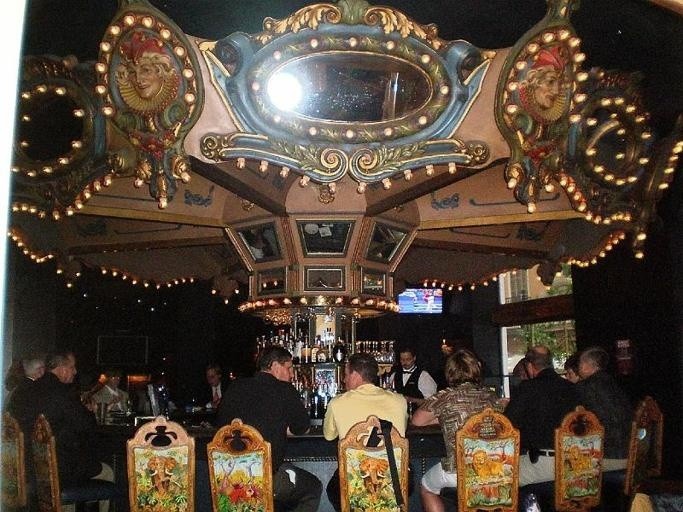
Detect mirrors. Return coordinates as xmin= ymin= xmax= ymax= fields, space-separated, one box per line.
xmin=265 ymin=51 xmax=434 ymax=123
xmin=591 ymin=108 xmax=638 ymax=177
xmin=19 ymin=95 xmax=81 ymax=161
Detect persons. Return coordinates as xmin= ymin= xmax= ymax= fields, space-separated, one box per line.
xmin=513 ymin=358 xmax=529 ymax=380
xmin=201 ymin=364 xmax=233 ymax=410
xmin=210 ymin=347 xmax=323 ymax=512
xmin=389 ymin=348 xmax=436 ymax=418
xmin=31 ymin=350 xmax=114 ymax=512
xmin=8 ymin=352 xmax=44 ymax=413
xmin=324 ymin=352 xmax=407 ymax=511
xmin=411 ymin=351 xmax=500 ymax=511
xmin=504 ymin=346 xmax=583 ymax=487
xmin=573 ymin=350 xmax=636 ymax=471
xmin=564 ymin=355 xmax=581 ymax=383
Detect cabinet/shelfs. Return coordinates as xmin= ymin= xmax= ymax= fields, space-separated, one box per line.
xmin=258 ymin=362 xmax=396 ymax=421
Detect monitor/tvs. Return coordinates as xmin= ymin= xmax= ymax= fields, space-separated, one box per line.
xmin=396 ymin=287 xmax=444 ymax=315
xmin=96 ymin=334 xmax=149 ymax=368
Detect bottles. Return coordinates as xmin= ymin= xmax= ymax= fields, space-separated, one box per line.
xmin=293 ymin=328 xmax=303 ymax=365
xmin=310 ymin=387 xmax=322 ymax=419
xmin=378 ymin=373 xmax=392 ymax=391
xmin=255 ymin=326 xmax=293 ymax=365
xmin=354 ymin=339 xmax=398 ymax=364
xmin=310 ymin=342 xmax=320 ymax=364
xmin=300 ymin=335 xmax=310 ymax=365
xmin=332 ymin=337 xmax=344 ymax=363
xmin=408 ymin=401 xmax=414 ymax=431
xmin=319 ymin=383 xmax=331 ymax=419
xmin=499 ymin=385 xmax=505 ymax=399
xmin=315 ymin=341 xmax=327 ymax=364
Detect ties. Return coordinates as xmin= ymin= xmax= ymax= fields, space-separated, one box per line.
xmin=214 ymin=389 xmax=219 ymax=403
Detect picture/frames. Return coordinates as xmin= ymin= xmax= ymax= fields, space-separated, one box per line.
xmin=360 ymin=268 xmax=387 ymax=295
xmin=363 ymin=222 xmax=408 ymax=262
xmin=236 ymin=220 xmax=284 ymax=263
xmin=257 ymin=266 xmax=285 ymax=297
xmin=303 ymin=265 xmax=346 ymax=292
xmin=296 ymin=219 xmax=355 ymax=258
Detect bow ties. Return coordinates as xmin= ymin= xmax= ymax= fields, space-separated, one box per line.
xmin=402 ymin=370 xmax=410 ymax=375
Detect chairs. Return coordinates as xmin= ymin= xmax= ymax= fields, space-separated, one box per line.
xmin=625 ymin=395 xmax=661 ymax=495
xmin=455 ymin=407 xmax=520 ymax=512
xmin=555 ymin=406 xmax=604 ymax=511
xmin=207 ymin=419 xmax=272 ymax=512
xmin=31 ymin=415 xmax=116 ymax=512
xmin=1 ymin=411 xmax=25 ymax=504
xmin=339 ymin=415 xmax=408 ymax=512
xmin=126 ymin=416 xmax=195 ymax=512
